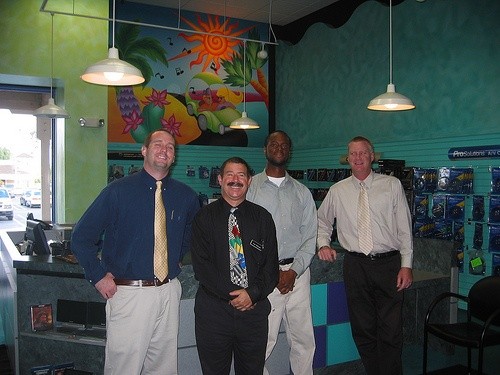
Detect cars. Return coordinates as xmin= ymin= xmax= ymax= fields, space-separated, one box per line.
xmin=0 ymin=186 xmax=15 ymax=221
xmin=20 ymin=189 xmax=54 ymax=208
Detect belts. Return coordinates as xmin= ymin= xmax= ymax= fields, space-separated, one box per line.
xmin=112 ymin=278 xmax=170 ymax=287
xmin=346 ymin=249 xmax=400 ymax=261
xmin=277 ymin=257 xmax=294 ymax=266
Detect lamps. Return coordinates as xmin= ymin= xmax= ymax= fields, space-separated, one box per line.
xmin=80 ymin=118 xmax=104 ymax=128
xmin=229 ymin=40 xmax=260 ymax=129
xmin=369 ymin=0 xmax=415 ymax=111
xmin=81 ymin=0 xmax=145 ymax=86
xmin=33 ymin=13 xmax=70 ymax=117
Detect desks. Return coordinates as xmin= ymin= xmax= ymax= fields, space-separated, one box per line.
xmin=407 ymin=269 xmax=449 ymax=340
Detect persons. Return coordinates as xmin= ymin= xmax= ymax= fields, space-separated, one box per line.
xmin=246 ymin=130 xmax=317 ymax=375
xmin=189 ymin=157 xmax=279 ymax=375
xmin=317 ymin=136 xmax=414 ymax=375
xmin=71 ymin=130 xmax=202 ymax=375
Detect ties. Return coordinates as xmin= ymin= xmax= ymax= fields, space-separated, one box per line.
xmin=152 ymin=182 xmax=169 ymax=283
xmin=356 ymin=183 xmax=373 ymax=256
xmin=227 ymin=207 xmax=249 ymax=289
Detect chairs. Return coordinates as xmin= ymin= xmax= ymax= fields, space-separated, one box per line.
xmin=422 ymin=276 xmax=500 ymax=375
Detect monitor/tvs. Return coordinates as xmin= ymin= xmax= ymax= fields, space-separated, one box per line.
xmin=25 ymin=220 xmax=51 ymax=255
